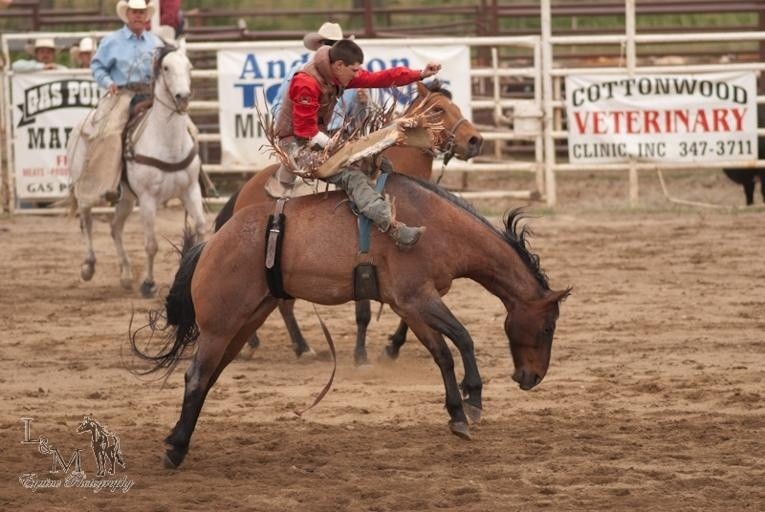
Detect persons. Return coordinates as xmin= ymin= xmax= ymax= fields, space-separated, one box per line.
xmin=71 ymin=40 xmax=100 ymax=68
xmin=145 ymin=1 xmax=184 ymax=38
xmin=277 ymin=38 xmax=443 ymax=250
xmin=12 ymin=36 xmax=71 ymax=209
xmin=272 ymin=21 xmax=376 ymax=151
xmin=90 ymin=0 xmax=216 ymax=200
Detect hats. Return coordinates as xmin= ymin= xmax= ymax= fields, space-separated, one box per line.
xmin=304 ymin=22 xmax=355 ymax=51
xmin=116 ymin=0 xmax=157 ymax=23
xmin=25 ymin=37 xmax=67 ymax=58
xmin=69 ymin=37 xmax=99 ymax=64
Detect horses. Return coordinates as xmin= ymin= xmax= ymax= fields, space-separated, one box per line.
xmin=59 ymin=30 xmax=208 ymax=300
xmin=719 ymin=75 xmax=764 ymax=207
xmin=120 ymin=78 xmax=576 ymax=471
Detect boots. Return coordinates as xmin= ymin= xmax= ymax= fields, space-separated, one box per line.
xmin=363 ymin=200 xmax=427 ymax=253
